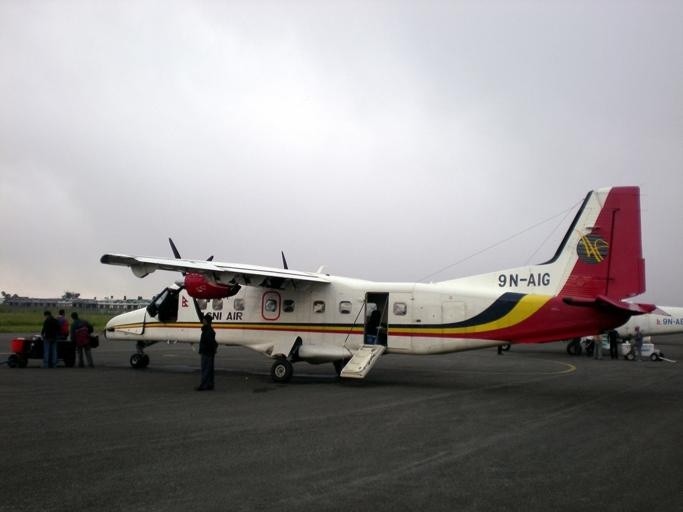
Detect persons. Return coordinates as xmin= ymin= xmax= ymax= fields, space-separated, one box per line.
xmin=193 ymin=315 xmax=219 ymax=391
xmin=633 ymin=325 xmax=643 ymax=361
xmin=581 ymin=336 xmax=595 ymax=357
xmin=566 ymin=337 xmax=582 ymax=354
xmin=68 ymin=312 xmax=96 ymax=368
xmin=607 ymin=329 xmax=619 ymax=359
xmin=55 ymin=309 xmax=69 ymax=341
xmin=365 ymin=310 xmax=384 ymax=345
xmin=40 ymin=310 xmax=60 ymax=368
xmin=590 ymin=334 xmax=603 ymax=360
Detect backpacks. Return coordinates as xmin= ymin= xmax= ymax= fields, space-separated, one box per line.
xmin=76 ymin=327 xmax=89 ymax=347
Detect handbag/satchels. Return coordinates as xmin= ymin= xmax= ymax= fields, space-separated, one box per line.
xmin=89 ymin=335 xmax=99 ymax=348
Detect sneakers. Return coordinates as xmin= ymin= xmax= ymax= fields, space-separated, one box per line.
xmin=193 ymin=384 xmax=215 ymax=391
